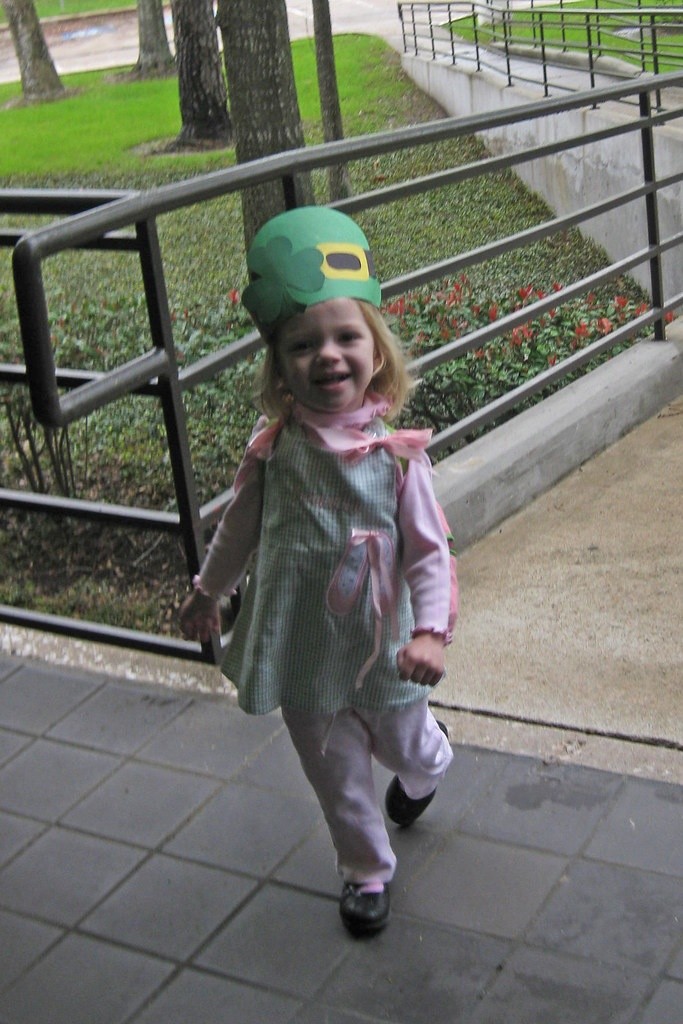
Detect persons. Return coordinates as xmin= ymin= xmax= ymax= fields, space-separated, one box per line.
xmin=178 ymin=264 xmax=453 ymax=941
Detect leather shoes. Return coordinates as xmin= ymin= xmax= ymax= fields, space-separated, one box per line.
xmin=341 ymin=880 xmax=393 ymax=938
xmin=386 ymin=719 xmax=449 ymax=824
